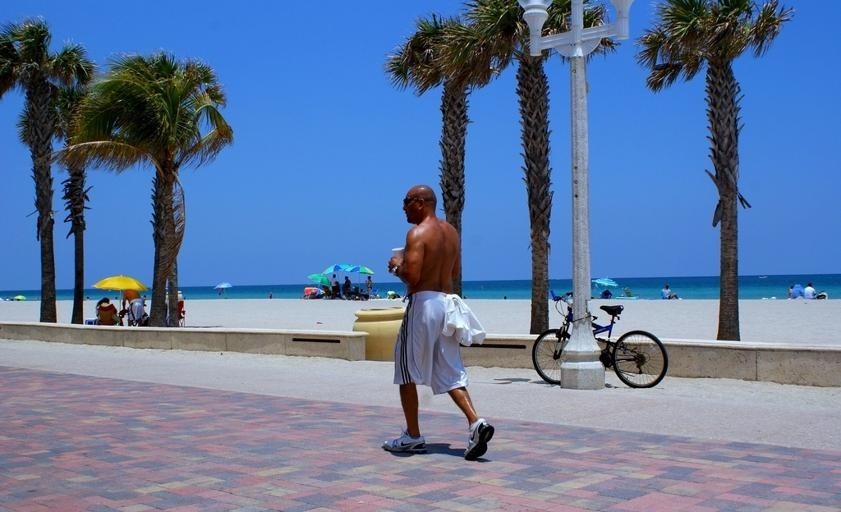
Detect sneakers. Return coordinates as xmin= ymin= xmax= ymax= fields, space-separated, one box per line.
xmin=464 ymin=421 xmax=494 ymax=460
xmin=381 ymin=430 xmax=426 ymax=451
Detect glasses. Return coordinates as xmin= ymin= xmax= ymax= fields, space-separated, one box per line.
xmin=403 ymin=197 xmax=415 ymax=203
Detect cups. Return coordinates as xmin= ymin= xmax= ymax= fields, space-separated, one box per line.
xmin=392 ymin=247 xmax=405 ymax=260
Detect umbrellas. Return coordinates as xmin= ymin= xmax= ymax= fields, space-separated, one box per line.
xmin=591 ymin=277 xmax=619 ymax=288
xmin=213 ymin=281 xmax=232 ymax=289
xmin=344 ymin=264 xmax=375 ymax=293
xmin=14 ymin=294 xmax=26 ymax=301
xmin=89 ymin=273 xmax=149 ymax=326
xmin=323 ymin=264 xmax=351 ymax=275
xmin=306 ymin=273 xmax=332 ymax=288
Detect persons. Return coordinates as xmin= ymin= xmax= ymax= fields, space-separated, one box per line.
xmin=322 ymin=285 xmax=330 ymax=298
xmin=123 ymin=290 xmax=144 ymax=325
xmin=344 ymin=276 xmax=352 ymax=298
xmin=331 ymin=273 xmax=340 ymax=299
xmin=96 ymin=296 xmax=117 ymax=326
xmin=661 ymin=284 xmax=682 ymax=300
xmin=269 ymin=292 xmax=273 ymax=299
xmin=600 ymin=289 xmax=613 ymax=298
xmin=623 ymin=286 xmax=633 ymax=297
xmin=366 ymin=275 xmax=373 ymax=300
xmin=382 ymin=184 xmax=495 ymax=461
xmin=787 ymin=280 xmax=829 ymax=300
xmin=219 ymin=288 xmax=224 ymax=296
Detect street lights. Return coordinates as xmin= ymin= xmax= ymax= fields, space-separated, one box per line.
xmin=517 ymin=0 xmax=635 ymax=389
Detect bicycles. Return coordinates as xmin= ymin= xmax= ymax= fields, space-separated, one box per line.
xmin=531 ymin=296 xmax=668 ymax=389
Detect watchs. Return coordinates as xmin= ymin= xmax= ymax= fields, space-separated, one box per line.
xmin=390 ymin=264 xmax=401 ymax=275
